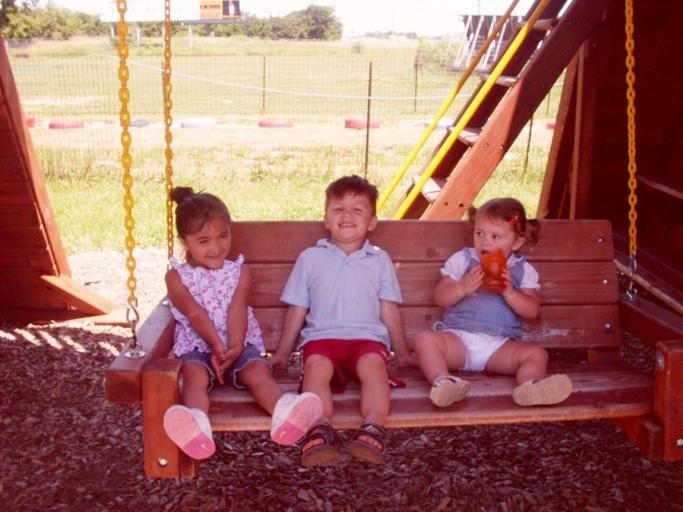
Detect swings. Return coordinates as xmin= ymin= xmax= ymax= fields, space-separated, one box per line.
xmin=104 ymin=1 xmax=683 ymax=480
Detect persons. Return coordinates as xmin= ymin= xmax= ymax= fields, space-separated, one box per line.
xmin=413 ymin=198 xmax=573 ymax=407
xmin=163 ymin=186 xmax=323 ymax=461
xmin=268 ymin=175 xmax=419 ymax=467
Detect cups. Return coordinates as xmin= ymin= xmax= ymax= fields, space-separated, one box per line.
xmin=479 ymin=246 xmax=509 ymax=295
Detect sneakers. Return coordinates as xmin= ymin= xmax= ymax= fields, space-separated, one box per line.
xmin=162 ymin=404 xmax=216 ymax=462
xmin=427 ymin=375 xmax=472 ymax=409
xmin=510 ymin=372 xmax=575 ymax=410
xmin=269 ymin=390 xmax=324 ymax=446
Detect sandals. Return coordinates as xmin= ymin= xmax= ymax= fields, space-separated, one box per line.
xmin=298 ymin=418 xmax=344 ymax=470
xmin=345 ymin=419 xmax=391 ymax=468
